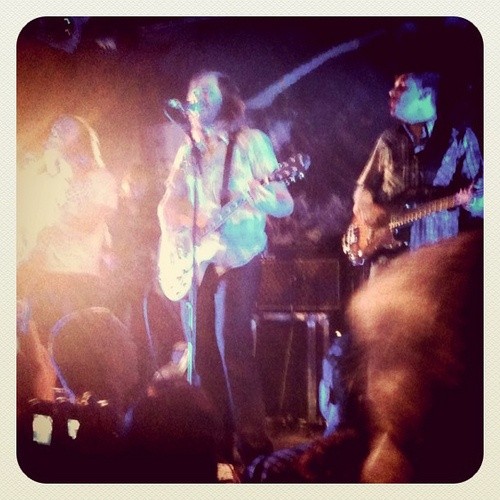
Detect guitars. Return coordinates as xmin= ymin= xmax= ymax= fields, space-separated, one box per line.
xmin=342 ymin=184 xmax=475 ymax=264
xmin=158 ymin=153 xmax=312 ymax=303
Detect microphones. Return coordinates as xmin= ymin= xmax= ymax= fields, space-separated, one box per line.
xmin=168 ymin=98 xmax=201 ymax=116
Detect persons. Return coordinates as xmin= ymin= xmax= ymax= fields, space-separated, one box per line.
xmin=30 ymin=112 xmax=111 ymax=351
xmin=245 ymin=334 xmax=382 ymax=483
xmin=111 ymin=166 xmax=163 ymax=383
xmin=19 ymin=299 xmax=245 ymax=484
xmin=155 ymin=72 xmax=291 ymax=454
xmin=343 ymin=236 xmax=484 ymax=485
xmin=351 ymin=70 xmax=482 ymax=288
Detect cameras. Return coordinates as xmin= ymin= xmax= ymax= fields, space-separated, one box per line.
xmin=28 ymin=399 xmax=116 ymax=464
